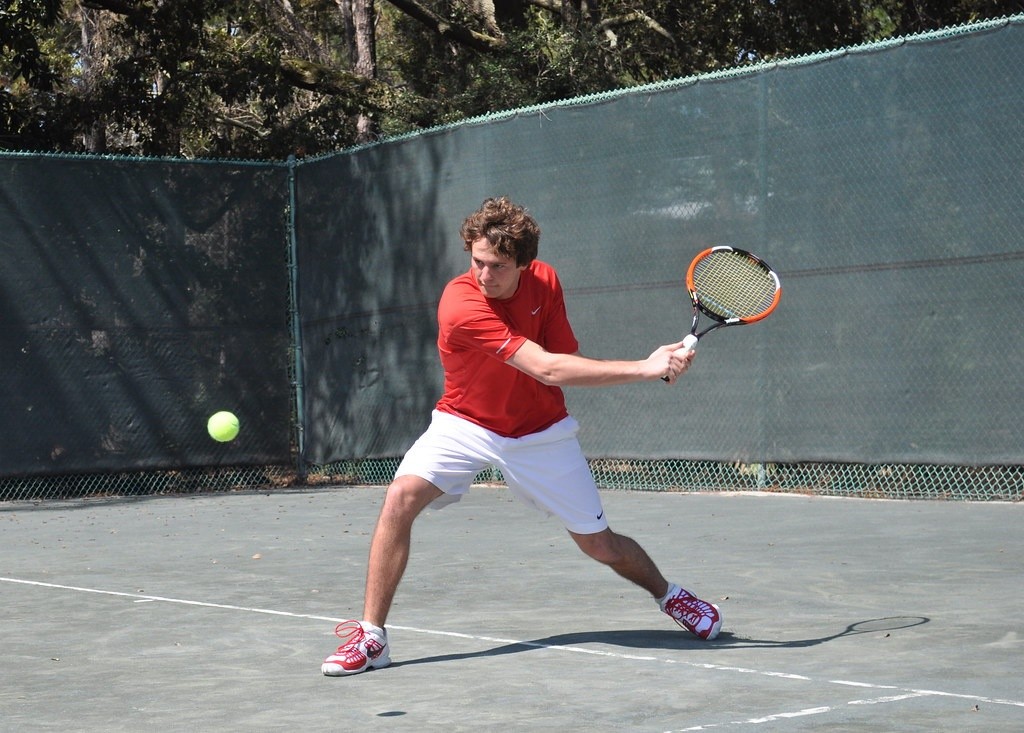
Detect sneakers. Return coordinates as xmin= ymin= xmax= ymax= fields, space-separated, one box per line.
xmin=660 ymin=585 xmax=722 ymax=640
xmin=321 ymin=620 xmax=391 ymax=676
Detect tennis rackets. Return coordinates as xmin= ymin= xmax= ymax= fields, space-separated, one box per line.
xmin=661 ymin=244 xmax=781 ymax=382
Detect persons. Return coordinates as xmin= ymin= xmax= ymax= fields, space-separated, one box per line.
xmin=321 ymin=195 xmax=724 ymax=676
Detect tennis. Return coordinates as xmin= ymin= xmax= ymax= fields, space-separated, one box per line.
xmin=207 ymin=410 xmax=239 ymax=442
